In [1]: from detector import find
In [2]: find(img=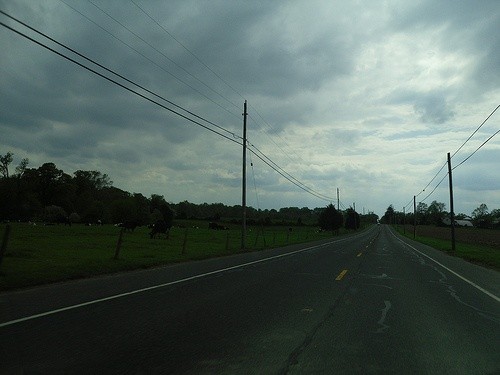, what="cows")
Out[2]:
[5,216,225,239]
